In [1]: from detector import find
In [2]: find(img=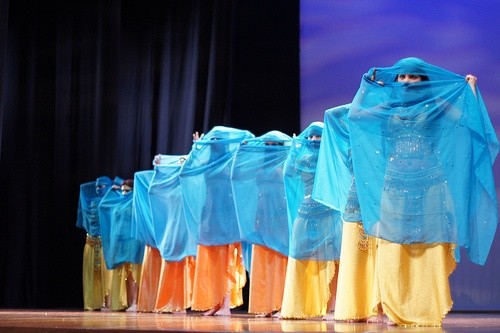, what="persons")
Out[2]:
[274,122,337,321]
[348,57,478,326]
[178,126,259,318]
[77,176,123,314]
[230,129,294,318]
[96,179,134,310]
[131,171,157,312]
[324,102,392,324]
[148,153,192,312]
